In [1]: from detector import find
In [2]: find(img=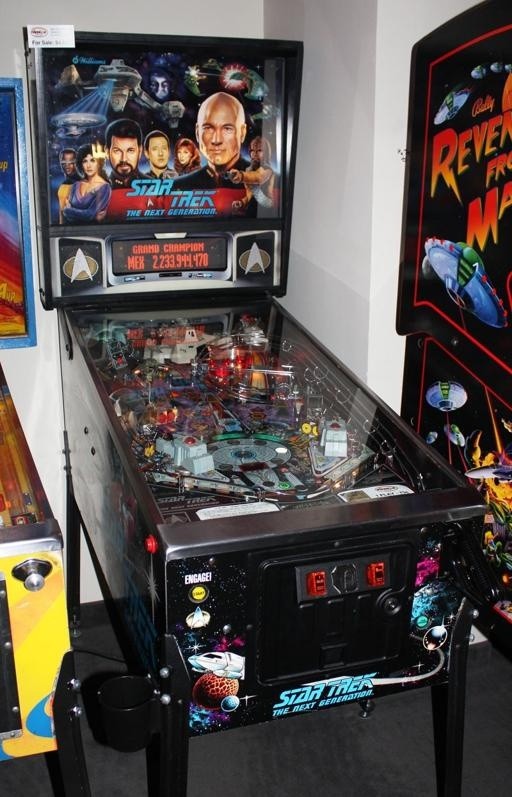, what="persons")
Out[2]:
[225,133,278,217]
[143,127,179,179]
[61,141,113,225]
[56,148,82,223]
[160,91,280,190]
[103,118,154,193]
[172,137,202,177]
